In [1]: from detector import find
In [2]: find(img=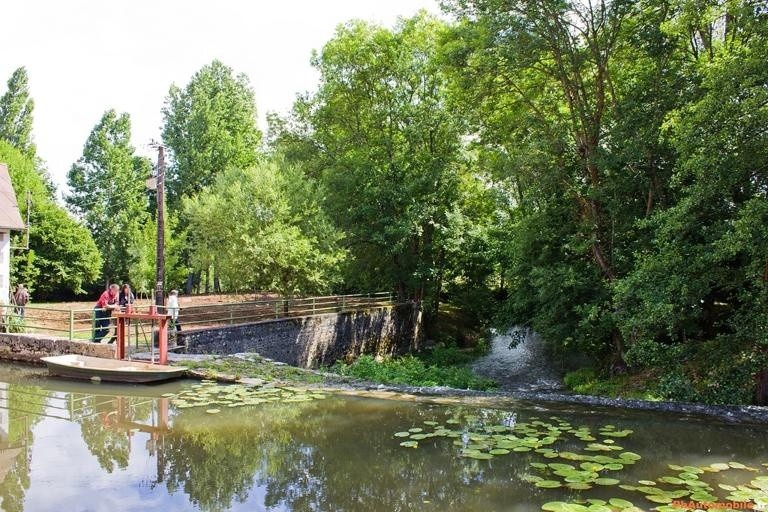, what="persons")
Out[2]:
[163,289,182,332]
[12,283,28,320]
[91,284,119,343]
[108,284,135,344]
